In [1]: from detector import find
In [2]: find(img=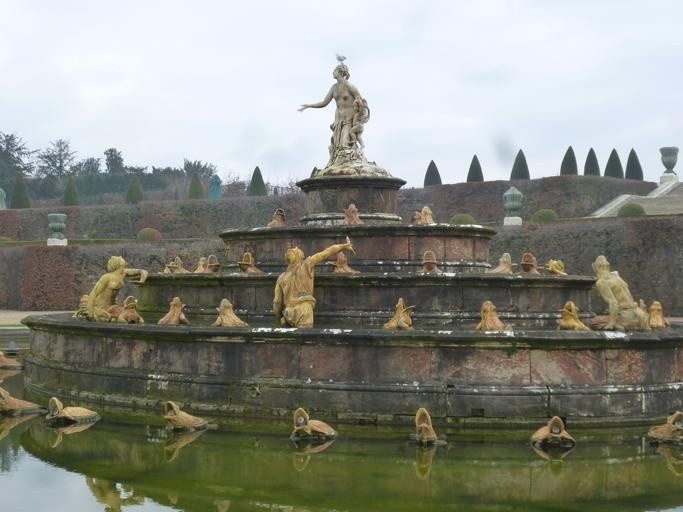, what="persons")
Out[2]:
[271,242,357,328]
[589,254,647,330]
[297,62,370,149]
[86,254,149,323]
[349,98,366,151]
